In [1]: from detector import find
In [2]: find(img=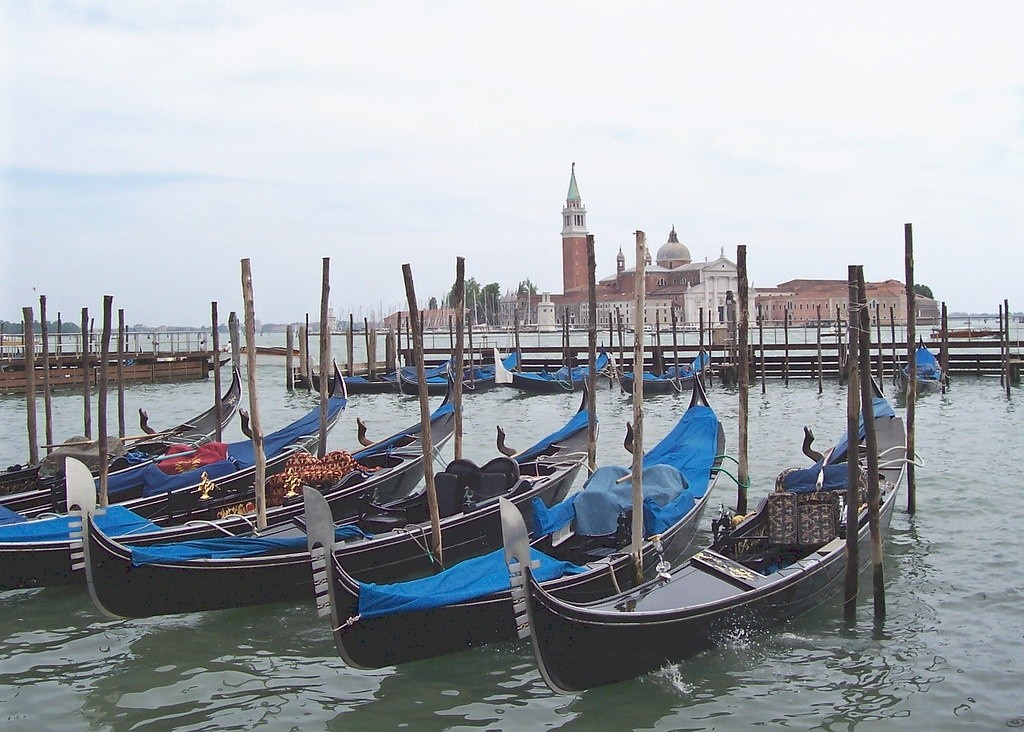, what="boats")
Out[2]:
[299,371,727,678]
[0,372,464,594]
[609,344,711,396]
[492,339,610,391]
[900,334,943,393]
[496,371,908,698]
[65,375,600,621]
[929,327,1007,338]
[298,351,403,395]
[394,347,522,399]
